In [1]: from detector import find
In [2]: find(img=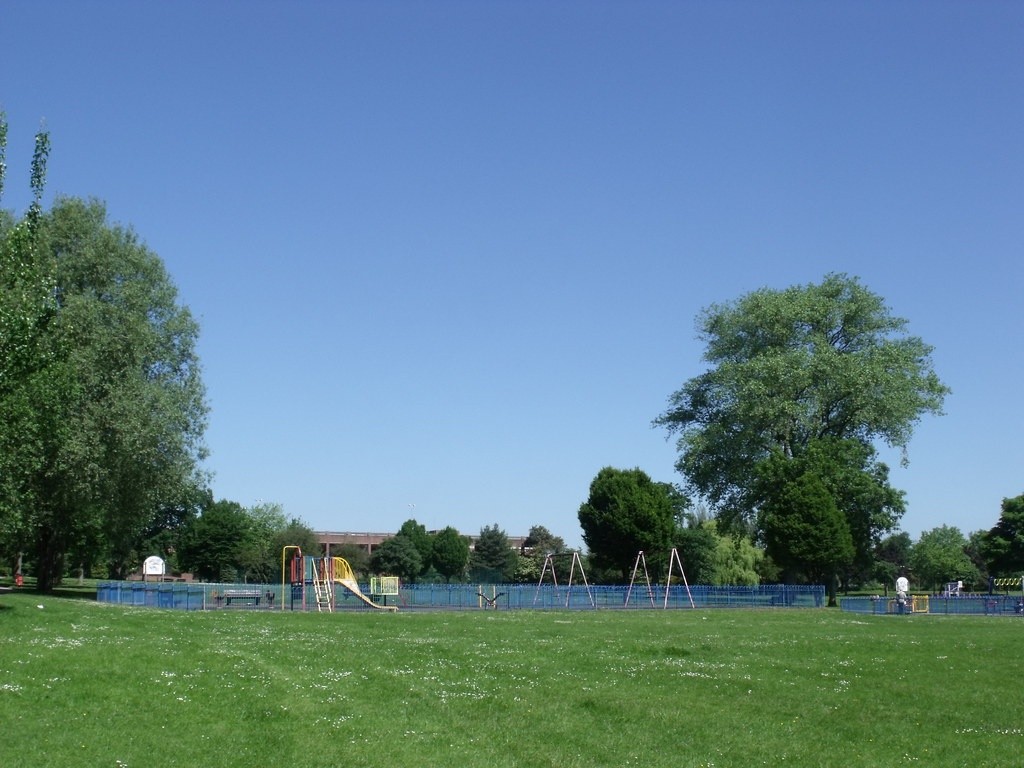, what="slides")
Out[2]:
[335,577,400,613]
